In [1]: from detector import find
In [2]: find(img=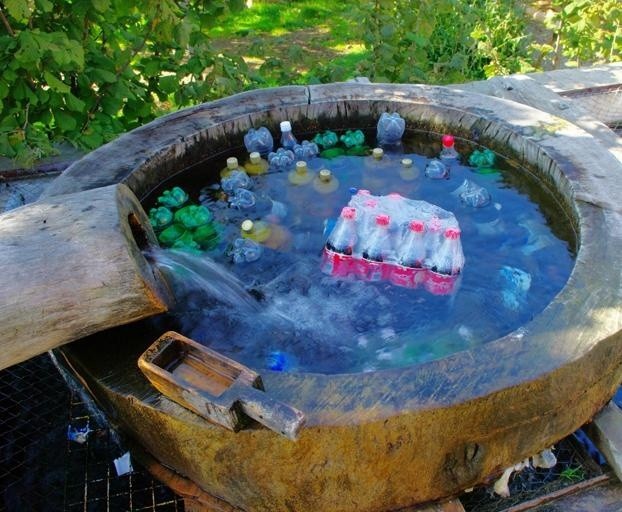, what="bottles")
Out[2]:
[138,111,557,322]
